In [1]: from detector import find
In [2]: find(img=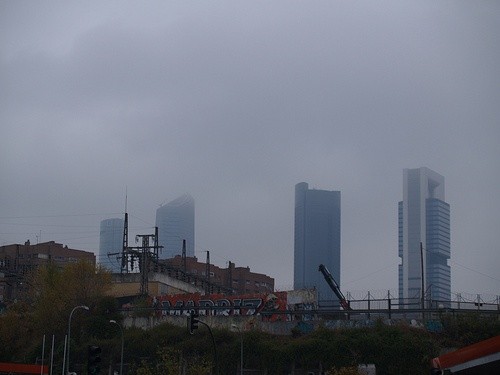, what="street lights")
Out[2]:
[231,324,244,375]
[109,318,125,375]
[193,317,218,375]
[66,304,90,375]
